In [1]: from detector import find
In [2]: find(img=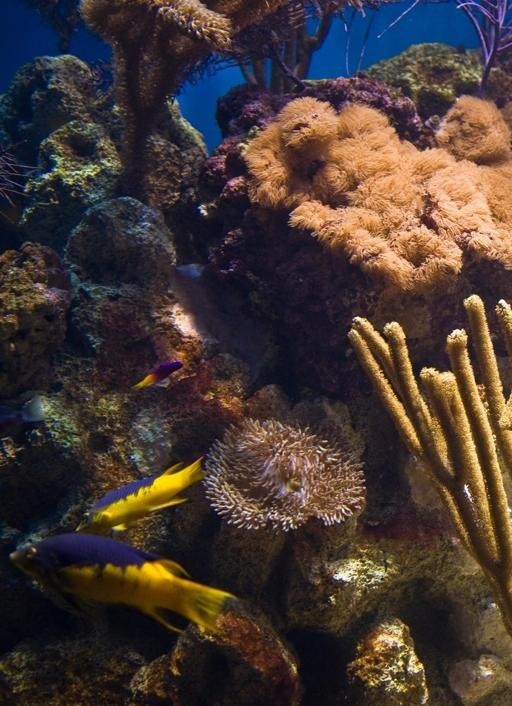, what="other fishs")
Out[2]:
[7,531,237,635]
[131,362,184,390]
[69,456,210,532]
